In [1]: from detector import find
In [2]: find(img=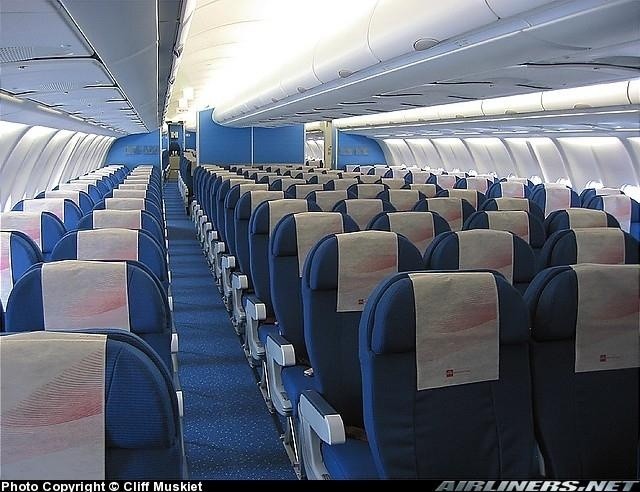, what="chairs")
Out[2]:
[177,147,640,481]
[0,163,183,481]
